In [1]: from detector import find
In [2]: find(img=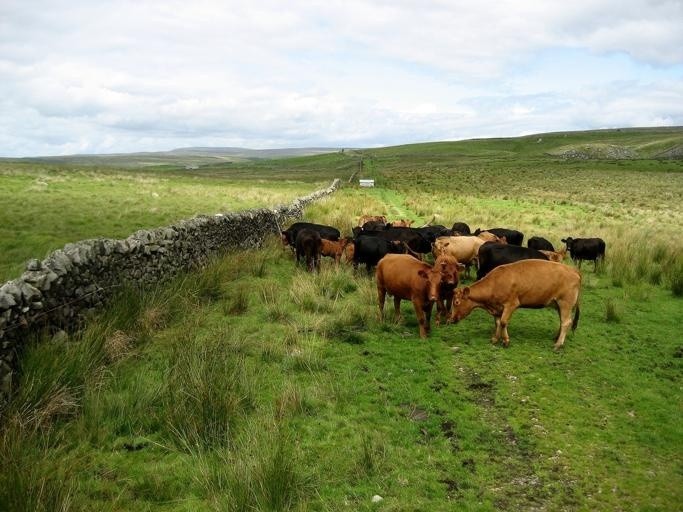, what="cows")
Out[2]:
[561,237,605,273]
[321,236,355,273]
[282,222,340,260]
[345,215,568,340]
[450,259,582,349]
[288,228,322,275]
